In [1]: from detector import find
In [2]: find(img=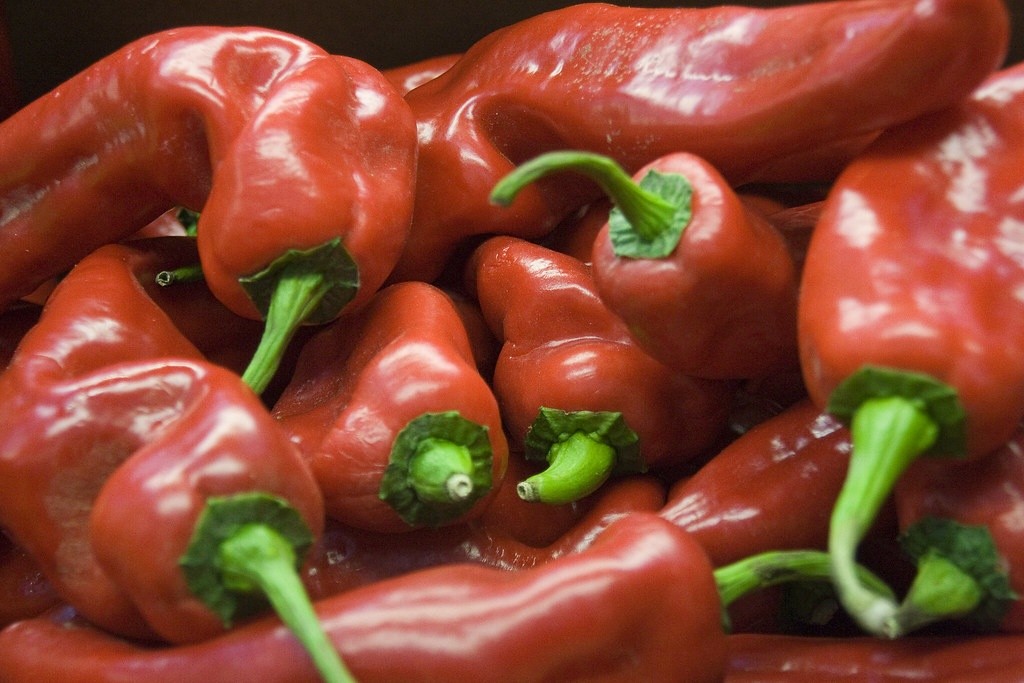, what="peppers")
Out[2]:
[0,0,1024,683]
